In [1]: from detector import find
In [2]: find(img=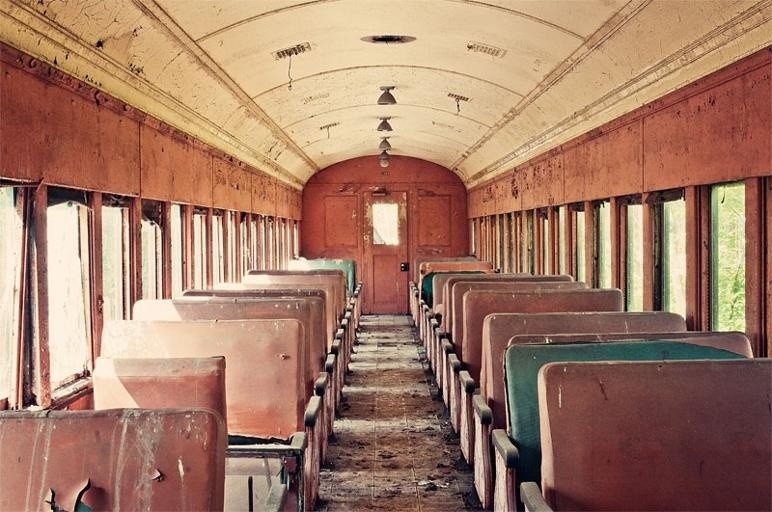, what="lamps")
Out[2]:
[375,86,398,169]
